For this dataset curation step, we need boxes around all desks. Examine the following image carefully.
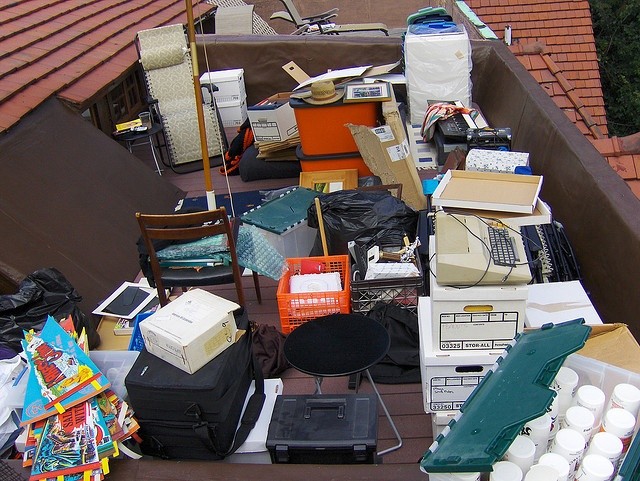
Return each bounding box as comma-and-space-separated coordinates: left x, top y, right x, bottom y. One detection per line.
282, 312, 402, 456
113, 118, 171, 177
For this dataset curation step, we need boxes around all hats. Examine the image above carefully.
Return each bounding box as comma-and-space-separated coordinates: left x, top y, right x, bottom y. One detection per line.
297, 80, 347, 104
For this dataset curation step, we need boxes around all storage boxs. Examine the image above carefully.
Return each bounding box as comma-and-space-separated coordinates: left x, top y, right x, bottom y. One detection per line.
350, 243, 425, 321
247, 90, 300, 143
417, 318, 640, 477
199, 68, 248, 103
430, 411, 462, 446
521, 280, 604, 331
140, 287, 246, 374
417, 296, 506, 412
229, 184, 325, 280
83, 351, 139, 404
292, 86, 388, 154
277, 255, 350, 336
300, 167, 358, 200
295, 143, 404, 177
524, 323, 640, 376
266, 394, 378, 465
429, 235, 528, 347
202, 98, 248, 126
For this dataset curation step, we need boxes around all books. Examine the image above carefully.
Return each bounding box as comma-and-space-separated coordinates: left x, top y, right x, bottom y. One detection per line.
18, 314, 142, 480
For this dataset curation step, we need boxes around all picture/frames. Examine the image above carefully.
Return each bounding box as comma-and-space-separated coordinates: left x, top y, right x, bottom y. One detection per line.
345, 81, 394, 102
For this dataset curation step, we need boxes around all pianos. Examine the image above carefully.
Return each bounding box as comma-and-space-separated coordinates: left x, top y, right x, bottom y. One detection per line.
427, 100, 478, 143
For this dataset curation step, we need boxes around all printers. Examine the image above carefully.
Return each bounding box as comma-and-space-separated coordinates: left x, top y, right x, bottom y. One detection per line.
435, 212, 579, 287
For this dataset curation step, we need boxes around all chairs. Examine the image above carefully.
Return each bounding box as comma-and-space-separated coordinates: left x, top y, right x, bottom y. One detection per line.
268, 0, 391, 38
133, 22, 228, 175
135, 205, 262, 311
